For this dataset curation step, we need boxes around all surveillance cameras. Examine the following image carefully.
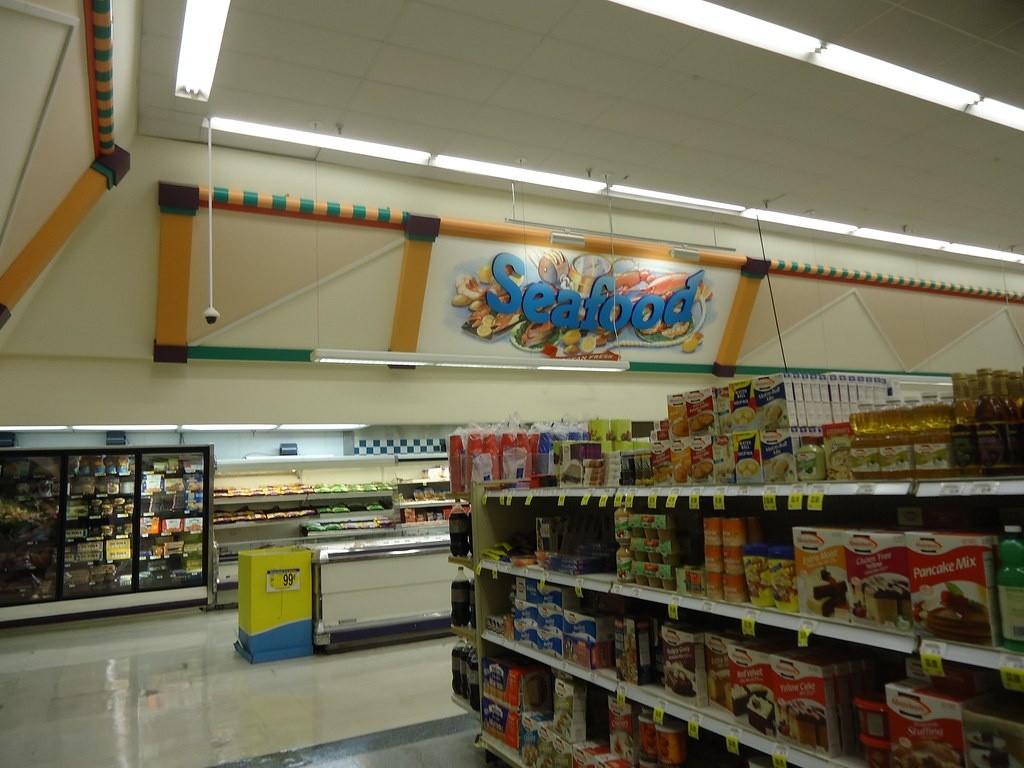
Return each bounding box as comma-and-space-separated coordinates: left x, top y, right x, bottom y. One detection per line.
203, 306, 220, 325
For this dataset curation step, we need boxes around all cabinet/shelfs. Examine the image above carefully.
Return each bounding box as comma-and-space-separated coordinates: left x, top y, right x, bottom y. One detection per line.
212, 453, 473, 609
442, 476, 1024, 768
0, 443, 215, 629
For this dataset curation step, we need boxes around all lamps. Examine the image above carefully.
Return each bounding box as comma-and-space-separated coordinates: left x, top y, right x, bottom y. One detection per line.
307, 146, 630, 374
201, 115, 1024, 266
174, 1, 233, 103
607, 0, 1024, 133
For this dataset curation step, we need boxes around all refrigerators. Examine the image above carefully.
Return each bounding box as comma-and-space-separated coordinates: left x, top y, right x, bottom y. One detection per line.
0, 444, 216, 629
301, 534, 451, 652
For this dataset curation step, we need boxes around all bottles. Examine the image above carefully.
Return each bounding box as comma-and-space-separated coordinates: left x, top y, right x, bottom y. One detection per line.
851, 397, 951, 480
950, 372, 977, 476
449, 498, 473, 557
622, 448, 654, 485
451, 567, 476, 630
997, 525, 1024, 653
975, 368, 1024, 476
452, 633, 480, 713
